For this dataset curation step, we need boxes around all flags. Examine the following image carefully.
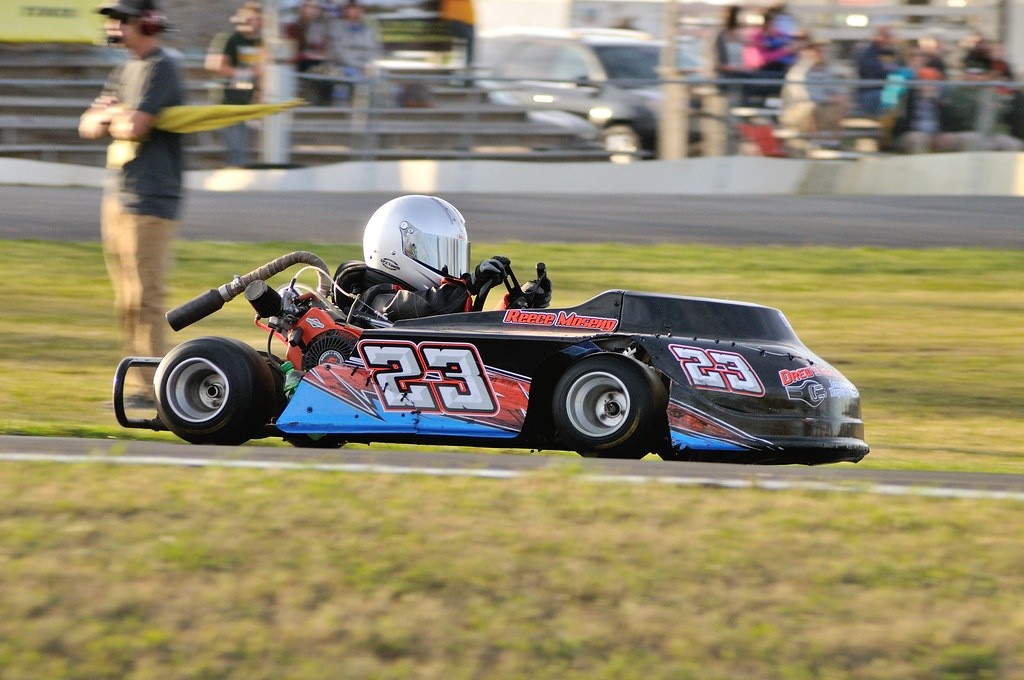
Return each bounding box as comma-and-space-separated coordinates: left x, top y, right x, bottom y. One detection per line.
152, 101, 292, 134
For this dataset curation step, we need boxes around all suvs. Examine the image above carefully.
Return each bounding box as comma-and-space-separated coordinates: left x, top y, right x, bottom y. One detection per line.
475, 28, 711, 148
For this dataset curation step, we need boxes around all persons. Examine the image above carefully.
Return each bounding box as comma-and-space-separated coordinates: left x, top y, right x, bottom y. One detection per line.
361, 193, 551, 320
286, 0, 330, 96
713, 0, 857, 148
205, 2, 265, 169
855, 27, 1013, 132
329, 0, 376, 106
78, 0, 187, 409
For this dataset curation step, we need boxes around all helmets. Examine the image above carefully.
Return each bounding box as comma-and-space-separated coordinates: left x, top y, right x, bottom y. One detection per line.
363, 193, 469, 292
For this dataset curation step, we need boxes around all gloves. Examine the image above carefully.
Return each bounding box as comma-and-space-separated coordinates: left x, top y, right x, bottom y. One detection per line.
462, 257, 510, 292
501, 275, 550, 310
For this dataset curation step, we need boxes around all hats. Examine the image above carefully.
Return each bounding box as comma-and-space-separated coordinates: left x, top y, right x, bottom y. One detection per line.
97, 0, 151, 16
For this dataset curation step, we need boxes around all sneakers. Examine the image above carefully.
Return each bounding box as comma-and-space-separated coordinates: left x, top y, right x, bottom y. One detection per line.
111, 393, 158, 410
